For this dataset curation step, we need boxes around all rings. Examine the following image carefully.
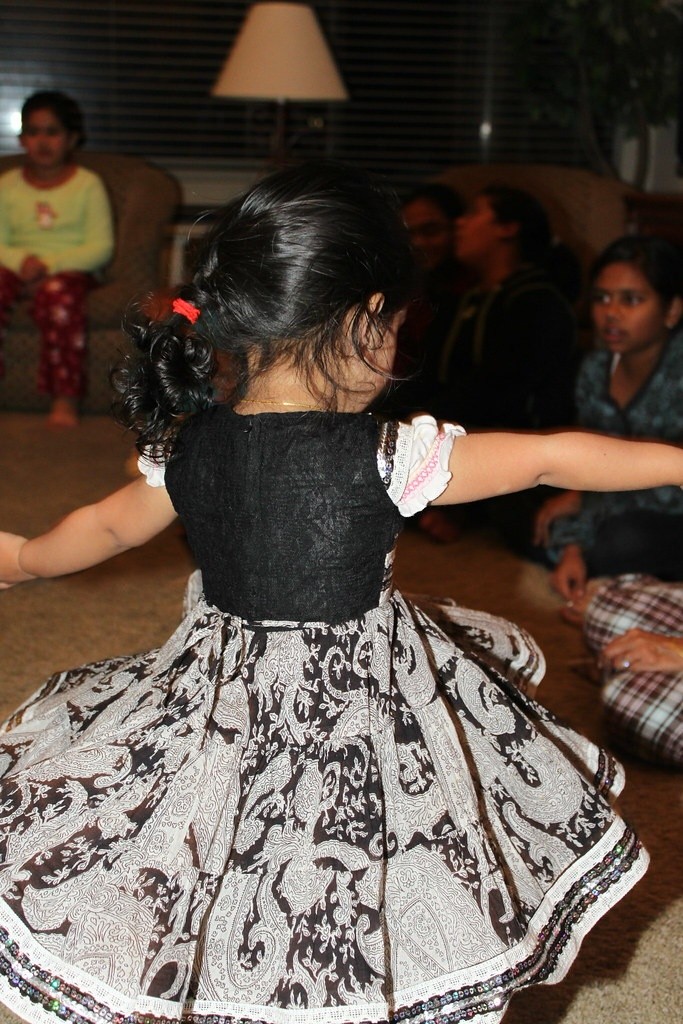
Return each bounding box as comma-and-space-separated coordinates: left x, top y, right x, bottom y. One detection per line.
622, 655, 629, 668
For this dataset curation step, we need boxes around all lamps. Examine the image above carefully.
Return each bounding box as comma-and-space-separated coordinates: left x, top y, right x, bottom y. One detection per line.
208, 1, 349, 169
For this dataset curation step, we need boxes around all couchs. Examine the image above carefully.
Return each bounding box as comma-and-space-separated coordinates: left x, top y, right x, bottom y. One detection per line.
0, 151, 180, 415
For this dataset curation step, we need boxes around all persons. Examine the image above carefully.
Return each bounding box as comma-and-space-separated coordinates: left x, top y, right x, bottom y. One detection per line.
0, 161, 682, 1024
353, 182, 683, 770
0, 91, 115, 432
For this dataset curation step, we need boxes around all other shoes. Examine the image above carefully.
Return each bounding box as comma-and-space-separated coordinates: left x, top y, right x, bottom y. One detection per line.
48, 410, 79, 428
